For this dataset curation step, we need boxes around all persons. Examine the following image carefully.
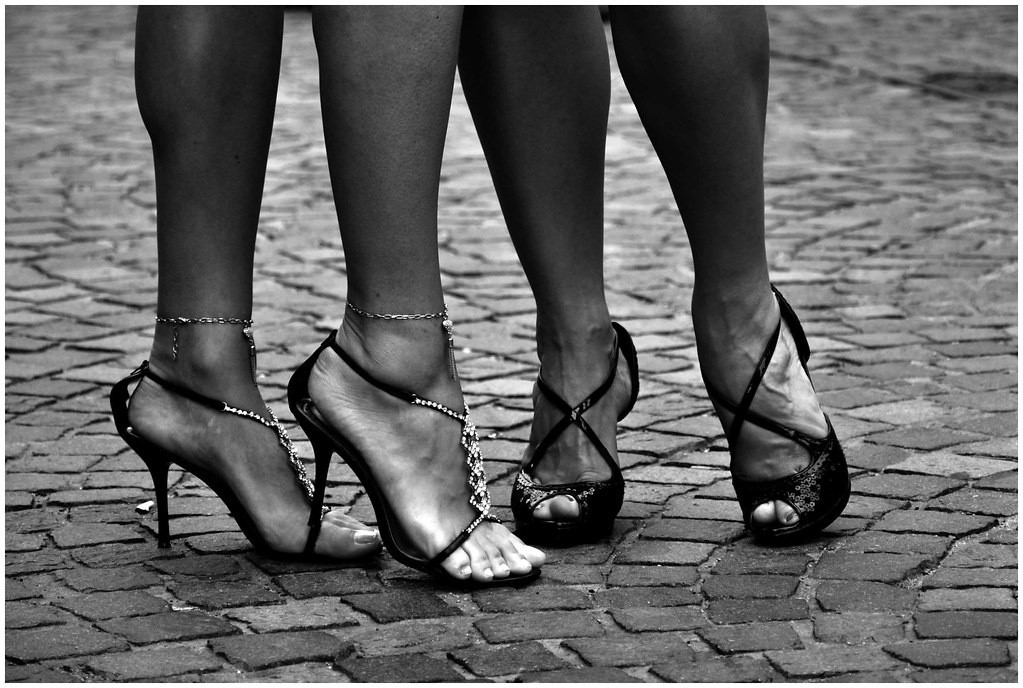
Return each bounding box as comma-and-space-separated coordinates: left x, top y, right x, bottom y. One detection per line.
107, 5, 546, 588
456, 5, 852, 545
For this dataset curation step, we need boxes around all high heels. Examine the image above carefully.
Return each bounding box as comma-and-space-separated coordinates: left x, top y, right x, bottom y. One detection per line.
701, 281, 851, 545
109, 350, 383, 562
287, 332, 541, 586
509, 322, 640, 545
